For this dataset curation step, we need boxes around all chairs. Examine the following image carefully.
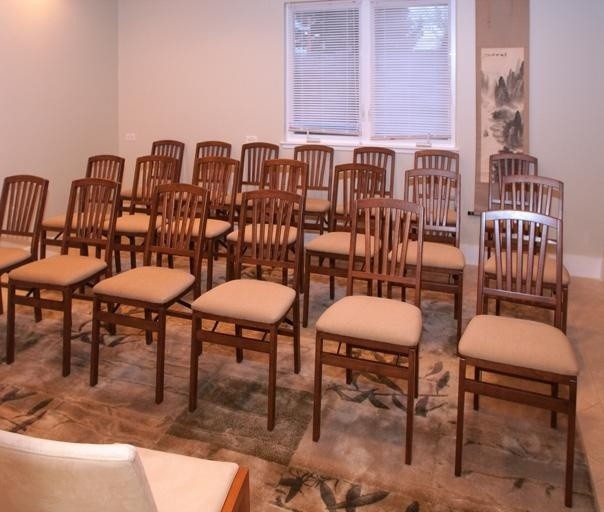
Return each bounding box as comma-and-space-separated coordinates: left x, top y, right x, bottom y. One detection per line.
41, 153, 124, 258
226, 158, 309, 293
388, 166, 466, 342
0, 430, 252, 512
0, 174, 50, 321
117, 139, 543, 254
303, 163, 386, 328
187, 190, 302, 432
310, 200, 427, 465
479, 173, 572, 333
156, 156, 244, 289
90, 183, 210, 404
455, 212, 578, 510
6, 178, 121, 377
95, 154, 180, 272
